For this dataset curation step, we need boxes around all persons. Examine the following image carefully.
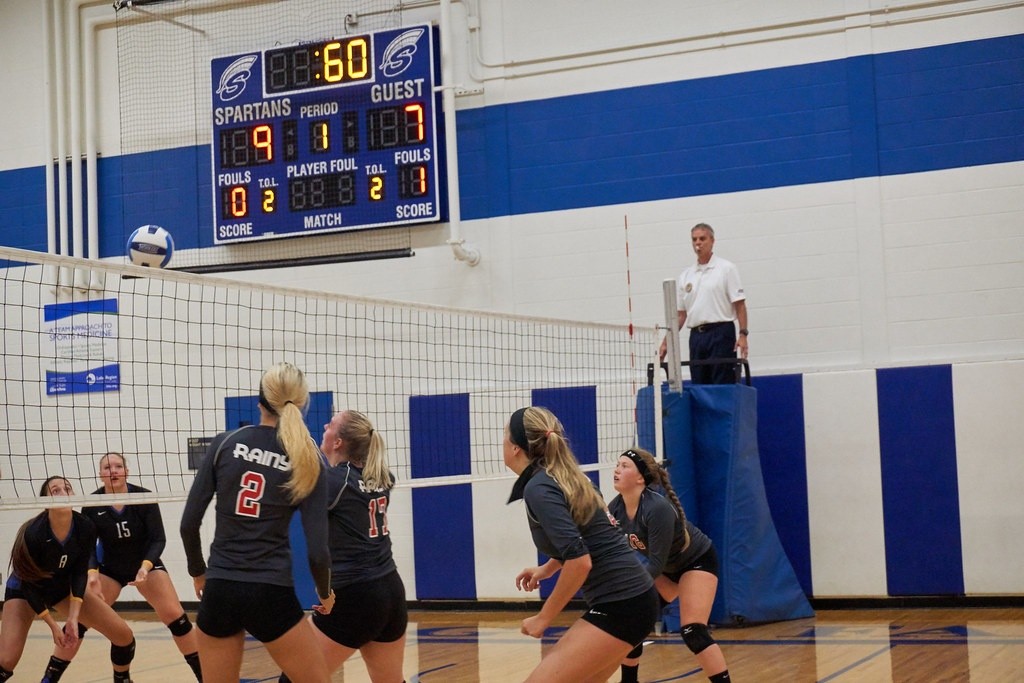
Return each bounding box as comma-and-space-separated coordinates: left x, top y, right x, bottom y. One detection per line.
180, 362, 335, 683
278, 410, 407, 683
504, 406, 660, 683
609, 449, 731, 683
659, 224, 748, 383
0, 475, 136, 683
40, 453, 202, 683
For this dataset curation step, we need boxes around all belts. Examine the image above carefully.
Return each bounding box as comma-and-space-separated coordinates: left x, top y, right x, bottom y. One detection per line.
692, 322, 733, 333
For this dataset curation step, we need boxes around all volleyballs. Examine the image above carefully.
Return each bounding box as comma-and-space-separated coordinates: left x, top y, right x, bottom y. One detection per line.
125, 224, 176, 270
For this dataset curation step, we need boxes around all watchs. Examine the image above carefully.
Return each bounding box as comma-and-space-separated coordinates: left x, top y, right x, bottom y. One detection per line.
740, 329, 748, 335
